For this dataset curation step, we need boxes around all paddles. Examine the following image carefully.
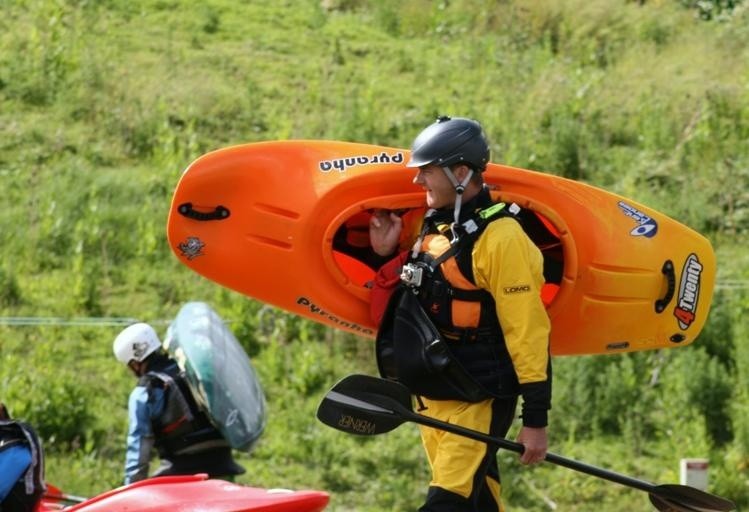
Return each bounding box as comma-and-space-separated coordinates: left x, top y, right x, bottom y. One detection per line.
42, 482, 88, 504
317, 374, 737, 512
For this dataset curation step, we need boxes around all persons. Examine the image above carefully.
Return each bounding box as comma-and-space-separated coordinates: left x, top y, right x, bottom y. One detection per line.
113, 322, 246, 486
365, 116, 553, 512
0, 402, 48, 511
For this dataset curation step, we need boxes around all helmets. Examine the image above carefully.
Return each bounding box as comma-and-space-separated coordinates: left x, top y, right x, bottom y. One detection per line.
112, 323, 163, 365
402, 115, 490, 173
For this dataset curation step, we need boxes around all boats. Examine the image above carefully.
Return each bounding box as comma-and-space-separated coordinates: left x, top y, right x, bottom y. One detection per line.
162, 301, 267, 450
165, 139, 717, 359
66, 473, 329, 512
